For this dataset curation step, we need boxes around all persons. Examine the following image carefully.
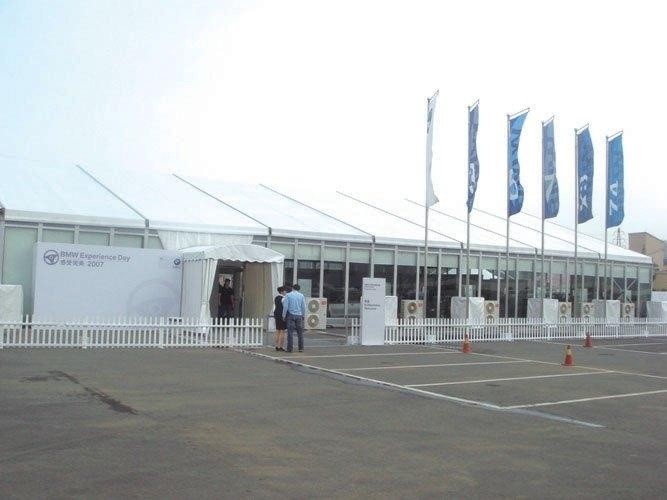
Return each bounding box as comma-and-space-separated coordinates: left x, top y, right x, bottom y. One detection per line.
273, 286, 288, 350
218, 278, 234, 330
281, 284, 305, 352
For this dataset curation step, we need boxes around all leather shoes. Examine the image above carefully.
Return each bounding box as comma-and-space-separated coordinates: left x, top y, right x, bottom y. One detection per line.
275, 346, 285, 351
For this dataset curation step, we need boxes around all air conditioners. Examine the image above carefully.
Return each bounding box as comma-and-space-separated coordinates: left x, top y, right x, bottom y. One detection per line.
619, 302, 635, 326
484, 301, 499, 328
399, 298, 426, 330
559, 301, 573, 328
304, 297, 327, 330
579, 302, 595, 326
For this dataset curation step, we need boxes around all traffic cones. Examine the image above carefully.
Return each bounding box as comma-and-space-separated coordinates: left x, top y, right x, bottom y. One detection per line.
583, 333, 593, 348
462, 334, 471, 353
562, 344, 573, 366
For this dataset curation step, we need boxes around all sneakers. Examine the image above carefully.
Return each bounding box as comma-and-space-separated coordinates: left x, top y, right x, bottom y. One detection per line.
284, 348, 291, 352
299, 349, 303, 352
